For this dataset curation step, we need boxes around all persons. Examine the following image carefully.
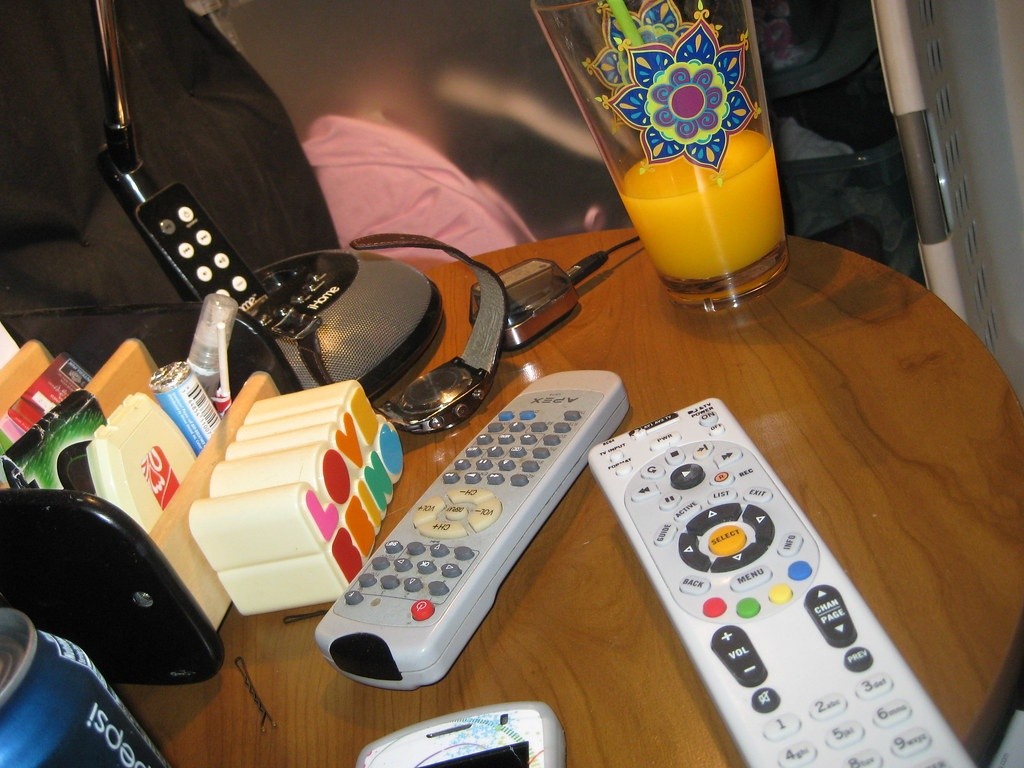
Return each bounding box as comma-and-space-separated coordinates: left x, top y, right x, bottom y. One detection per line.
0, 0, 929, 401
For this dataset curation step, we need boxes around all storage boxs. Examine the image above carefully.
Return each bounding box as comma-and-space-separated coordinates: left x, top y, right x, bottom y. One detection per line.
0, 303, 311, 687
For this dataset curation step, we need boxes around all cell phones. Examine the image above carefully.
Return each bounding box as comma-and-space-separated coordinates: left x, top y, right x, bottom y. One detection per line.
356, 699, 566, 768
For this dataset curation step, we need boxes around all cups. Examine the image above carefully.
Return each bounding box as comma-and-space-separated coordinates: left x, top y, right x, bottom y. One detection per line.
531, 0, 790, 314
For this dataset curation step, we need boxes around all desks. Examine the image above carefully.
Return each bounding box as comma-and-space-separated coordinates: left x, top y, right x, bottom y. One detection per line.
109, 227, 1024, 768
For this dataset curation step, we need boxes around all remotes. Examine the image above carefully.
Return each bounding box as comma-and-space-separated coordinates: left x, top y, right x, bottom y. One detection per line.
133, 180, 269, 317
313, 369, 634, 691
588, 398, 980, 768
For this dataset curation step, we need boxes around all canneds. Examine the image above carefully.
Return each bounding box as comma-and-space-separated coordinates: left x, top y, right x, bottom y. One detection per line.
0, 607, 175, 768
